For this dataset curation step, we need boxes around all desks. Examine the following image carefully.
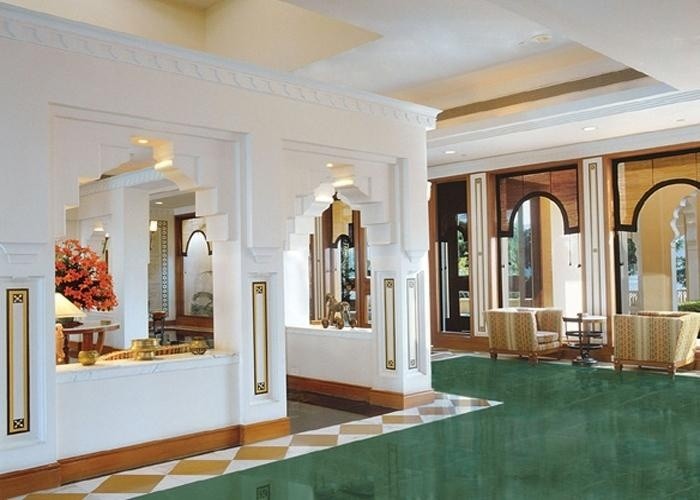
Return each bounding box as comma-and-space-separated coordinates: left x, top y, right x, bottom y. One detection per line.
63, 322, 120, 364
563, 314, 608, 367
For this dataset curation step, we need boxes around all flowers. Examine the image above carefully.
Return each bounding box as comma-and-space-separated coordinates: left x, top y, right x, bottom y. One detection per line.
55, 240, 119, 312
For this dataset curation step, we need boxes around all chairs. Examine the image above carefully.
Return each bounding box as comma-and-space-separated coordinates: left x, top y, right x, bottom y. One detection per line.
486, 307, 563, 364
614, 311, 700, 375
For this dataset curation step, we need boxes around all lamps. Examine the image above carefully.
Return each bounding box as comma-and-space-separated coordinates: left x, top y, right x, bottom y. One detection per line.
149, 221, 157, 250
54, 293, 88, 365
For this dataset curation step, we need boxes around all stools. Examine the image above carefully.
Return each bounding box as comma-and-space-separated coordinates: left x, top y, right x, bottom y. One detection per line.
152, 310, 167, 341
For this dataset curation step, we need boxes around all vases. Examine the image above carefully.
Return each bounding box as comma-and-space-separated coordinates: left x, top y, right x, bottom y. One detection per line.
56, 317, 73, 329
